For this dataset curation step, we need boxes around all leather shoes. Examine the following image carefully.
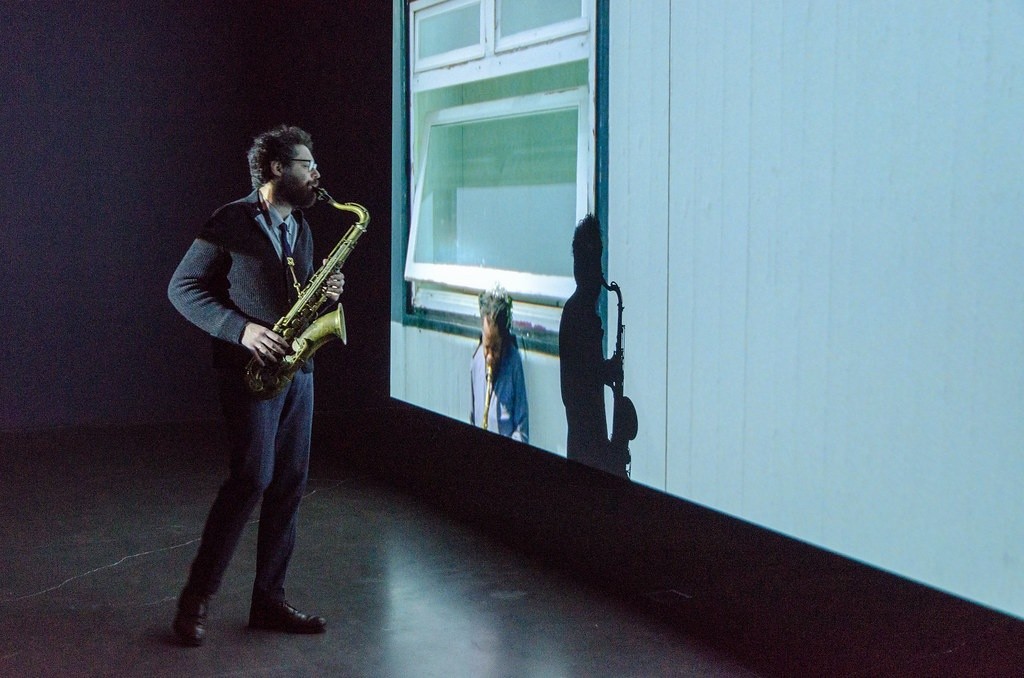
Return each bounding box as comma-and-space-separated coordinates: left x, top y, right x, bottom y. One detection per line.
172, 597, 209, 645
248, 604, 327, 633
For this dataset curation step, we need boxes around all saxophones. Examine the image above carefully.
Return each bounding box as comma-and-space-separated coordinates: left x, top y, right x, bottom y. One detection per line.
241, 187, 372, 402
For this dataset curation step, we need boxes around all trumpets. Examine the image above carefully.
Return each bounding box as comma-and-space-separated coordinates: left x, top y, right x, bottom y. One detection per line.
481, 355, 497, 431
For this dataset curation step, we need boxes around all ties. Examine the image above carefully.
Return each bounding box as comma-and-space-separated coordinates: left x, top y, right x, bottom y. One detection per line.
278, 222, 293, 263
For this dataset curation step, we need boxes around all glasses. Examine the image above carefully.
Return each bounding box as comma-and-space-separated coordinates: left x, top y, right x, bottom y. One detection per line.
288, 157, 318, 172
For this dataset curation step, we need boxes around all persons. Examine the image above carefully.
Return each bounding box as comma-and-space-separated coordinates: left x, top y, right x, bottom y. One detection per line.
166, 125, 347, 646
469, 284, 530, 444
